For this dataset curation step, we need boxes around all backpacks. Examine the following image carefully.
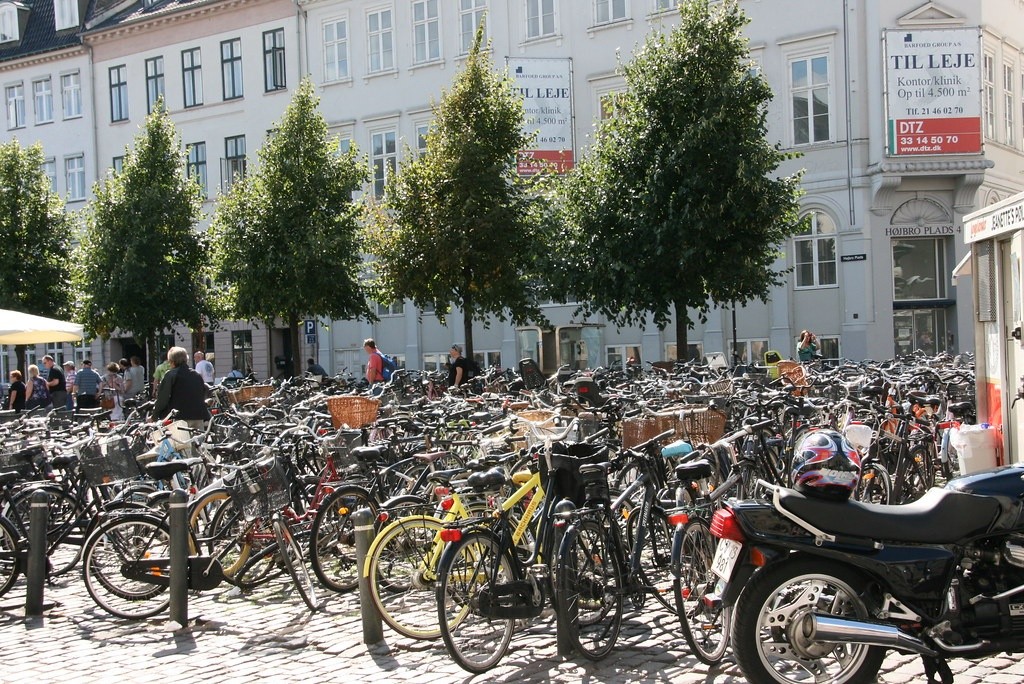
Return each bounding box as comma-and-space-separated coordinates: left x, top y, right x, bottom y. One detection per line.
373, 352, 398, 380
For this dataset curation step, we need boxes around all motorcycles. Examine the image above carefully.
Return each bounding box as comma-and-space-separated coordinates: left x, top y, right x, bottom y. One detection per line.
707, 379, 1023, 684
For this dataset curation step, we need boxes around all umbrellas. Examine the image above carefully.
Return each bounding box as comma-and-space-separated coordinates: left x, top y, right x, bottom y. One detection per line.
0, 309, 84, 345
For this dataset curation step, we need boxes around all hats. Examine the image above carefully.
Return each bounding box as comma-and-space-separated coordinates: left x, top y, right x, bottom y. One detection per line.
81, 360, 92, 366
62, 361, 74, 366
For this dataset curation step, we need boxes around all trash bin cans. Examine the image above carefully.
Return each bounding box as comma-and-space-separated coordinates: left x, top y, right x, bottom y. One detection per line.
950, 423, 997, 476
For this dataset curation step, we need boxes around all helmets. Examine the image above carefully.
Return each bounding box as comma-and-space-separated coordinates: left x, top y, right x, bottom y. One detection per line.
792, 429, 862, 500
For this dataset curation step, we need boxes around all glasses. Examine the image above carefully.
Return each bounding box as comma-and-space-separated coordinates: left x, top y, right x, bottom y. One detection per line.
451, 345, 457, 351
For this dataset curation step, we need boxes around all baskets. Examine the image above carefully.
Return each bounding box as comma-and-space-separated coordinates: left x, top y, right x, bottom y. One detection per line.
657, 403, 727, 449
227, 385, 272, 407
223, 453, 292, 523
622, 418, 658, 450
700, 380, 736, 396
327, 395, 378, 431
537, 441, 610, 507
79, 438, 141, 487
776, 361, 809, 396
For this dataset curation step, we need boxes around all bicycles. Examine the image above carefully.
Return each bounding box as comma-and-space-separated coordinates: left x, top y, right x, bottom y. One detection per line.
1, 353, 976, 677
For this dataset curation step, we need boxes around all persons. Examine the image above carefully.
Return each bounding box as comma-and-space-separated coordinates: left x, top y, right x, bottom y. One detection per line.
797, 330, 820, 396
147, 348, 207, 488
909, 328, 934, 353
305, 359, 328, 378
363, 338, 392, 438
444, 344, 468, 394
227, 364, 255, 382
194, 352, 214, 398
8, 357, 145, 428
